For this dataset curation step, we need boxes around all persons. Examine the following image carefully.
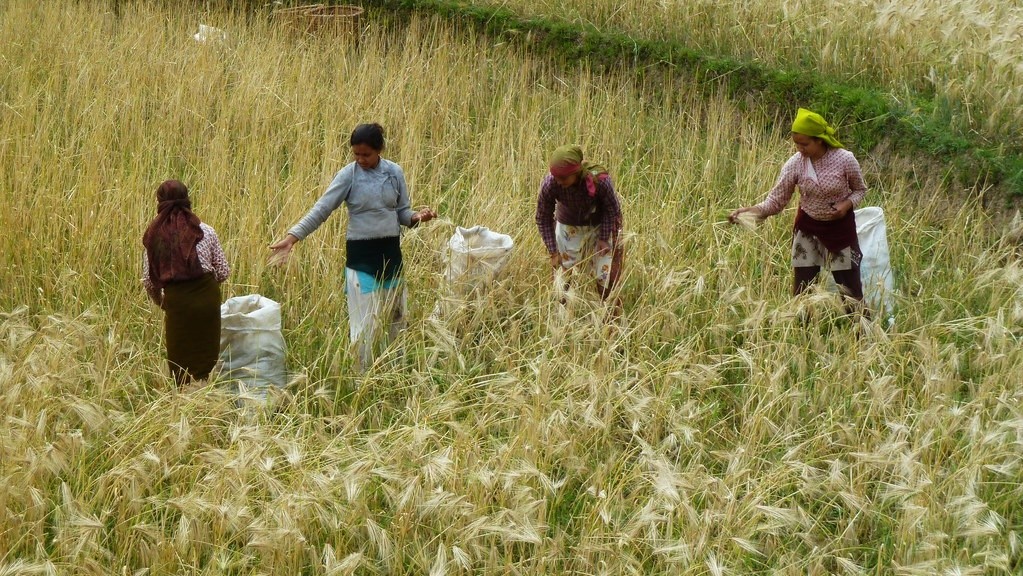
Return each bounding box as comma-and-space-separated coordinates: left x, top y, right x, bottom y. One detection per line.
729, 107, 870, 329
142, 180, 229, 387
535, 144, 626, 317
268, 122, 436, 368
